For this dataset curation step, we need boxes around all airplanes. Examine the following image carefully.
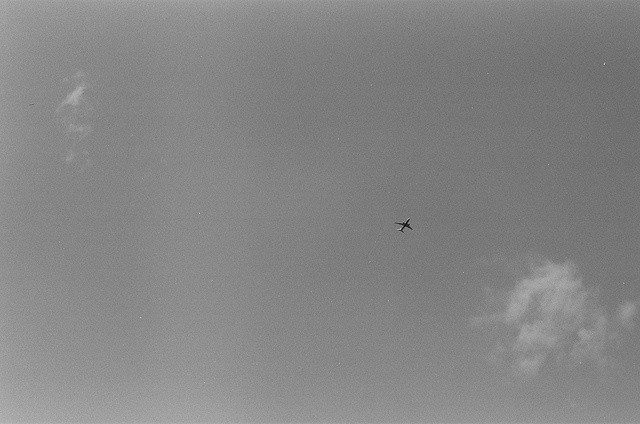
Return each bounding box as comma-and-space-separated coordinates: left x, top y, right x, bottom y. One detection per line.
395, 217, 413, 232
